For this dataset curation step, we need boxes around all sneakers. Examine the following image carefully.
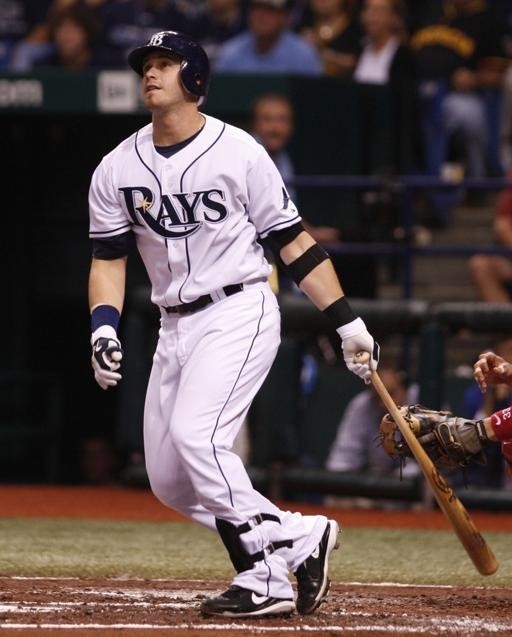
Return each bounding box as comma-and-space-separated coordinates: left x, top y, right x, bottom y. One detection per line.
295, 519, 340, 616
199, 585, 295, 618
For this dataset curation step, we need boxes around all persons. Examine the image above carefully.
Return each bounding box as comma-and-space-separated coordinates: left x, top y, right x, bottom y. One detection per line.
84, 30, 382, 618
2, 1, 512, 90
252, 45, 512, 510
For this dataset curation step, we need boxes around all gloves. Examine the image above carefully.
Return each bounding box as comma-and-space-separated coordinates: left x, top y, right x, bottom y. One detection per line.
90, 304, 125, 393
323, 297, 381, 385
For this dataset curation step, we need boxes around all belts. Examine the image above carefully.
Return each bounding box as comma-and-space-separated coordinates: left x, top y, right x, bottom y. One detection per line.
159, 282, 245, 318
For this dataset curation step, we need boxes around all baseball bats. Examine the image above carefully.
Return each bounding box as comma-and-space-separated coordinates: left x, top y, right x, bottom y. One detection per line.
354, 350, 500, 575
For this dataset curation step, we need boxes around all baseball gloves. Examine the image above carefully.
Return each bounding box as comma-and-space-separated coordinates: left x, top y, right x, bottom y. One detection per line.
379, 403, 501, 474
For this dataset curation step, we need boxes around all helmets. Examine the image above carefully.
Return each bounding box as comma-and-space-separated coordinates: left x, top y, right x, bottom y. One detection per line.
127, 28, 212, 108
